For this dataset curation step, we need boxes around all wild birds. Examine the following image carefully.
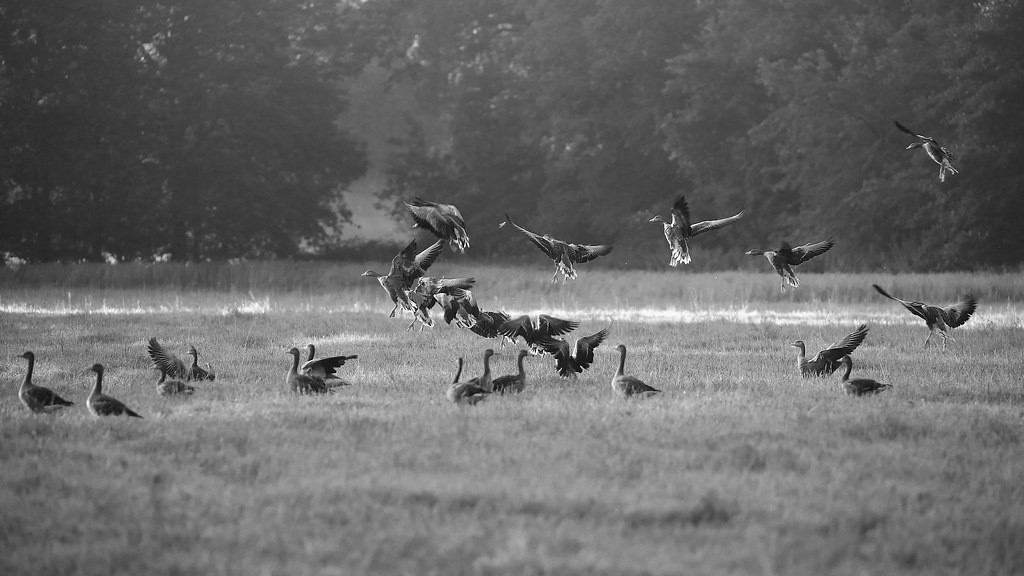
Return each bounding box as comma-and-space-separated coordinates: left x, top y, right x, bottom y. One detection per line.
788, 324, 870, 379
147, 335, 217, 384
498, 212, 616, 286
301, 343, 359, 389
836, 354, 894, 398
491, 349, 536, 395
15, 350, 76, 414
284, 346, 335, 394
744, 235, 836, 294
360, 195, 614, 380
445, 356, 493, 405
150, 362, 197, 397
648, 193, 745, 266
81, 363, 145, 419
467, 347, 502, 393
610, 343, 662, 398
872, 283, 978, 353
891, 117, 961, 183
